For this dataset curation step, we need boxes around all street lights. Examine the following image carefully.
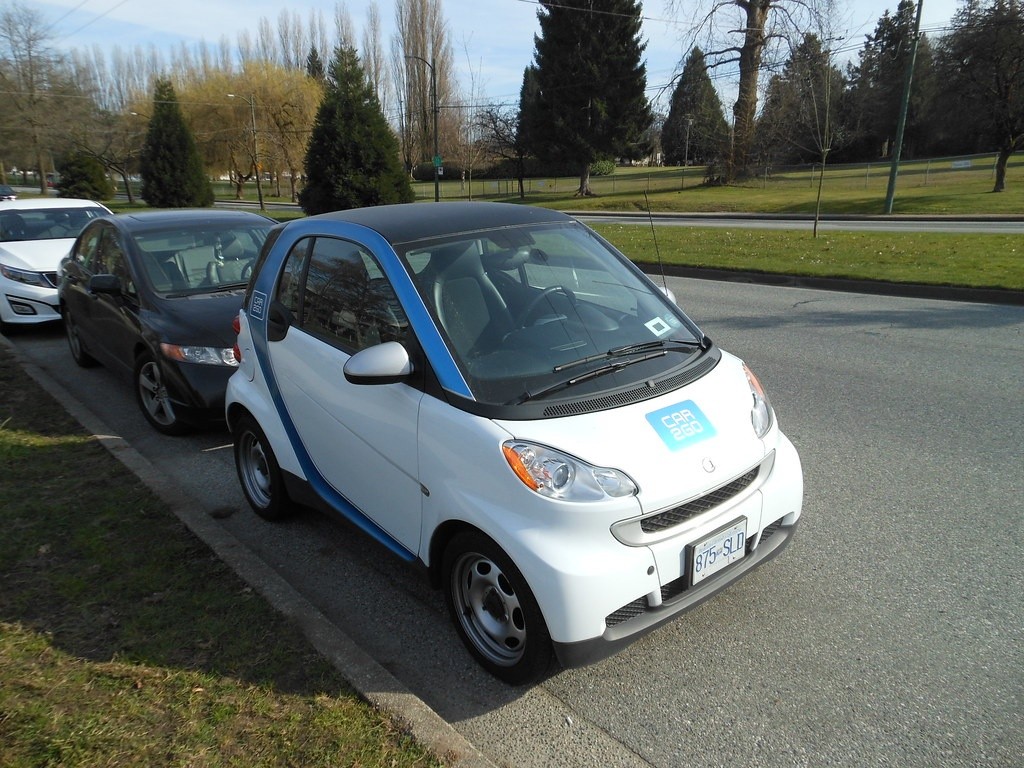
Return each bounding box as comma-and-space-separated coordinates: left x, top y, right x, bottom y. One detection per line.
402, 55, 437, 204
227, 94, 264, 210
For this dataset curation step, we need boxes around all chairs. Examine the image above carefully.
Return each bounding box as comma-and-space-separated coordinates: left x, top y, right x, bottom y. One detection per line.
101, 229, 258, 293
423, 240, 515, 363
305, 237, 401, 352
0, 212, 93, 240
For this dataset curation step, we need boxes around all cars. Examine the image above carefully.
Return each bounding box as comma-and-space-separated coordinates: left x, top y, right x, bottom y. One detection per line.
1, 196, 117, 326
223, 199, 804, 687
56, 208, 284, 431
